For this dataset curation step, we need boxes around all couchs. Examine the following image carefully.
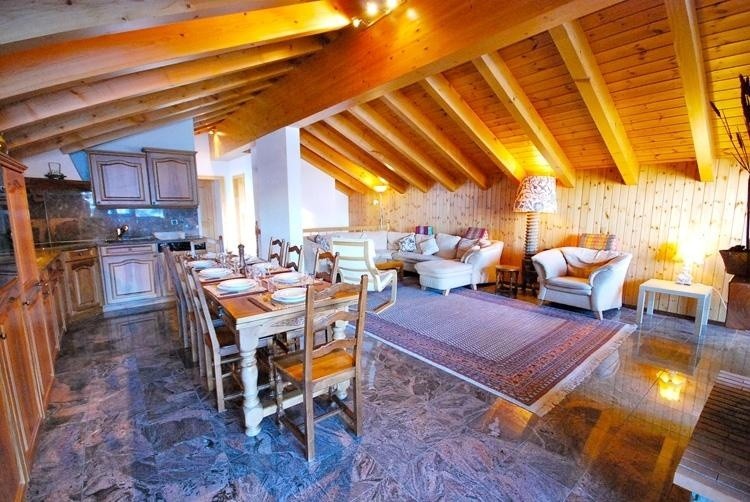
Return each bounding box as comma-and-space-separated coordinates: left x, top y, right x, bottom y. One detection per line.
305, 230, 503, 296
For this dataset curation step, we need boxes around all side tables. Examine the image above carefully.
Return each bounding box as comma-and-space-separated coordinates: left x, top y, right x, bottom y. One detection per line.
639, 279, 713, 336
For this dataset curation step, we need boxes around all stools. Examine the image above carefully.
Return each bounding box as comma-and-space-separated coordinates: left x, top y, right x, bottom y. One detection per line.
414, 261, 473, 296
496, 265, 520, 297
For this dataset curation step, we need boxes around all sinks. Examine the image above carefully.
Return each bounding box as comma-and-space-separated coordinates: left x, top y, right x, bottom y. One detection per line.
106, 236, 156, 244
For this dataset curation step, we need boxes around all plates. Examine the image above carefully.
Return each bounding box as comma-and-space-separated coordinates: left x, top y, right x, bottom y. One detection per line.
271, 272, 307, 303
233, 256, 257, 264
190, 260, 258, 291
200, 252, 219, 260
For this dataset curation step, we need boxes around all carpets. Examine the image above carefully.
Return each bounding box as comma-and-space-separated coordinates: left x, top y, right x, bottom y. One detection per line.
349, 275, 637, 416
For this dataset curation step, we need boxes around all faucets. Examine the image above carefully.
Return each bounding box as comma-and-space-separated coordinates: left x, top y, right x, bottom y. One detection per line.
116, 226, 129, 239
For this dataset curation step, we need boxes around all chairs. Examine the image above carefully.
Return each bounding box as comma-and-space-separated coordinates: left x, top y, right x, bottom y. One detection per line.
162, 247, 207, 349
267, 237, 285, 266
183, 260, 274, 414
312, 247, 340, 283
189, 236, 224, 254
269, 275, 366, 462
332, 238, 398, 315
283, 241, 304, 271
532, 247, 633, 321
170, 253, 221, 377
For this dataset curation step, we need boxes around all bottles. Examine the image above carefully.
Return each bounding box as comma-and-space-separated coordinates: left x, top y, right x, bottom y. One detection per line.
238, 244, 247, 272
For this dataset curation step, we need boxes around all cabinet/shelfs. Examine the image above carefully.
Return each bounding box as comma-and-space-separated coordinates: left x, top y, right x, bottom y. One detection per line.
141, 147, 198, 208
99, 246, 158, 314
0, 244, 101, 502
88, 150, 152, 207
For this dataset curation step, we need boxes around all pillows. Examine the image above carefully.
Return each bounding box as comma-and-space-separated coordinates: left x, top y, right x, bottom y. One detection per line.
369, 221, 492, 263
580, 233, 616, 250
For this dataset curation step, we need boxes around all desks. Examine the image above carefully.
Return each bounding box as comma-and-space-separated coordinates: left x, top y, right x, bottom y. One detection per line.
673, 370, 750, 502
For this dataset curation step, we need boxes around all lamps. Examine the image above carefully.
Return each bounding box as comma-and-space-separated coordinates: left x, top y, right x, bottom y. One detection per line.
514, 175, 557, 296
374, 186, 387, 230
